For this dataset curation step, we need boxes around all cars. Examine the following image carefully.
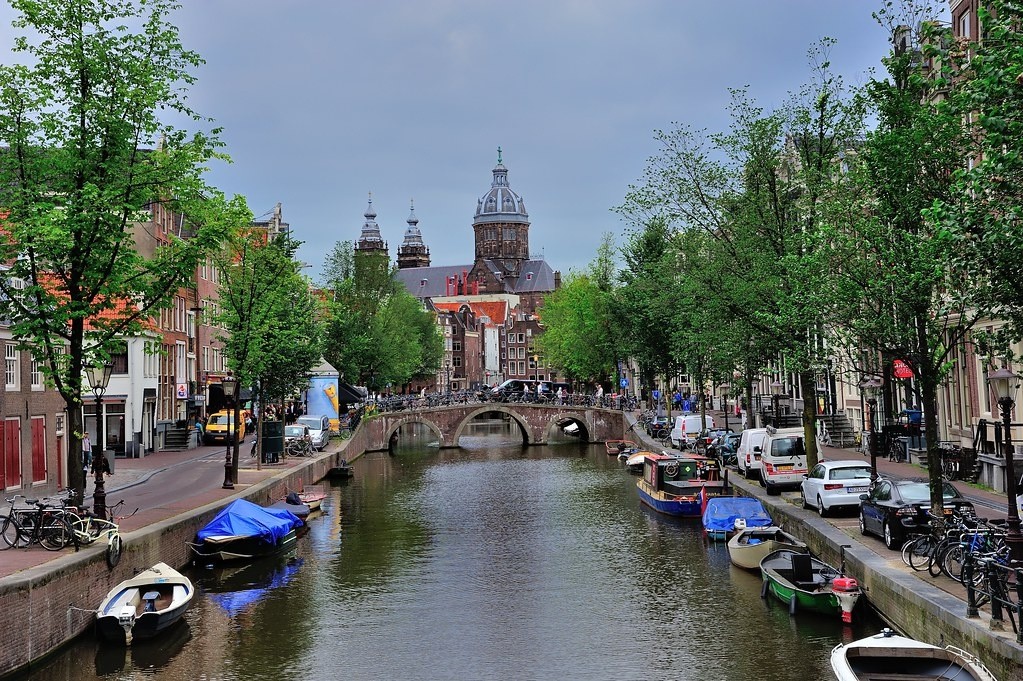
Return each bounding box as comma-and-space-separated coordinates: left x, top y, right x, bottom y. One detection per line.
220, 409, 255, 434
858, 475, 977, 553
695, 427, 741, 466
799, 460, 882, 517
285, 425, 311, 446
646, 415, 675, 439
205, 413, 246, 445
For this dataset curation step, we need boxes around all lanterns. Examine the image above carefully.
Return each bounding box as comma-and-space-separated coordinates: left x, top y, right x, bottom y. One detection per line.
486, 373, 490, 377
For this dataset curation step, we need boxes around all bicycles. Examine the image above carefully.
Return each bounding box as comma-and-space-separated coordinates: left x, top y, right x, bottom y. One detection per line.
863, 431, 911, 463
251, 432, 258, 457
286, 434, 320, 459
896, 500, 1023, 599
937, 444, 968, 483
0, 494, 140, 570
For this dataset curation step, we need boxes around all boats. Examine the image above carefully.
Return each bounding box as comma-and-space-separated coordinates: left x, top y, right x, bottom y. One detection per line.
602, 437, 732, 518
758, 547, 866, 619
720, 518, 810, 572
94, 561, 194, 646
333, 457, 356, 480
194, 497, 303, 568
829, 627, 997, 681
264, 478, 328, 524
701, 496, 778, 548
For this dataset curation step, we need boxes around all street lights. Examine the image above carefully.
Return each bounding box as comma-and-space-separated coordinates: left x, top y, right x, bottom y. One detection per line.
446, 362, 450, 406
217, 370, 244, 492
986, 365, 1023, 562
720, 382, 731, 436
82, 352, 120, 534
771, 379, 788, 434
861, 377, 883, 491
533, 353, 540, 401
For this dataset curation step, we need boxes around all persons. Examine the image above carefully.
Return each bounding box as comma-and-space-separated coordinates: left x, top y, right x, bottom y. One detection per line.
556, 387, 569, 406
674, 390, 683, 410
244, 401, 303, 435
595, 384, 603, 407
82, 432, 92, 467
522, 382, 530, 402
533, 381, 543, 403
421, 387, 426, 398
903, 405, 922, 422
196, 413, 210, 446
689, 394, 697, 412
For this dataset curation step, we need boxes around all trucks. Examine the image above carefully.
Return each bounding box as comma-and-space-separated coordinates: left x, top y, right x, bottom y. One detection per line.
670, 415, 716, 452
754, 424, 824, 496
735, 428, 766, 481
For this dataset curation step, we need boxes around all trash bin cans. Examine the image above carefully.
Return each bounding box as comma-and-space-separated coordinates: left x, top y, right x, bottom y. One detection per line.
102, 450, 114, 475
713, 396, 720, 410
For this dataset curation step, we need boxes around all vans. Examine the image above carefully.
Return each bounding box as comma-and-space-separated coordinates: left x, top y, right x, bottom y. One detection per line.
296, 414, 332, 450
489, 379, 555, 403
553, 383, 574, 404
